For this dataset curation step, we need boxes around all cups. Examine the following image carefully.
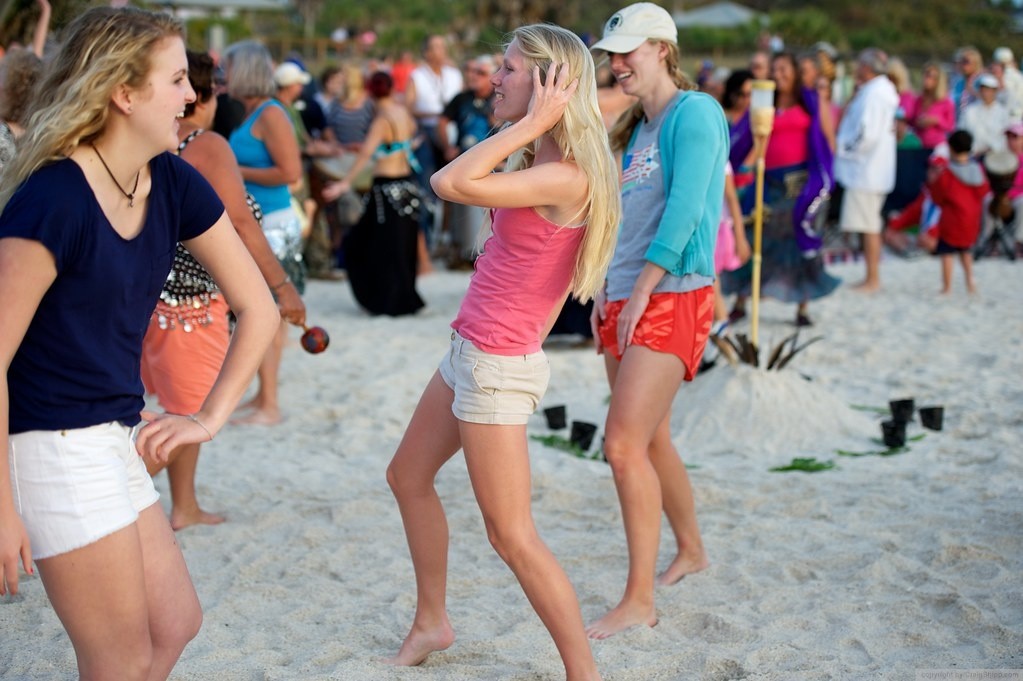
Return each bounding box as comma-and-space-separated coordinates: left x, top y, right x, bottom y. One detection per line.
544, 405, 566, 430
881, 398, 944, 449
571, 421, 598, 451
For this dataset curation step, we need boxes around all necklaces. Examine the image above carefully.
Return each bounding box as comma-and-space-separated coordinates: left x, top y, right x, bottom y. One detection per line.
90, 140, 139, 207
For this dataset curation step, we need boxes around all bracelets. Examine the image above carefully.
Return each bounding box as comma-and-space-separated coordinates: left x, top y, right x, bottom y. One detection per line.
190, 415, 214, 440
272, 277, 291, 290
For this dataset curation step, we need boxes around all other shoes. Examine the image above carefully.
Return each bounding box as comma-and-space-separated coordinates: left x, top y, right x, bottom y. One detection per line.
446, 257, 474, 270
727, 310, 744, 319
797, 313, 810, 326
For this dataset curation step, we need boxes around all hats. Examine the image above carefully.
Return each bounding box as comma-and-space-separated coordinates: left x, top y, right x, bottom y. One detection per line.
1004, 122, 1023, 136
925, 155, 949, 167
272, 62, 310, 86
590, 2, 677, 57
974, 74, 998, 89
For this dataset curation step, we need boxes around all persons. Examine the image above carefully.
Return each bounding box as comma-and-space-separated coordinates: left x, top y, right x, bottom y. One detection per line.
141, 50, 303, 530
586, 2, 729, 640
0, 8, 282, 680
386, 23, 619, 681
224, 39, 303, 426
0, 0, 1023, 341
